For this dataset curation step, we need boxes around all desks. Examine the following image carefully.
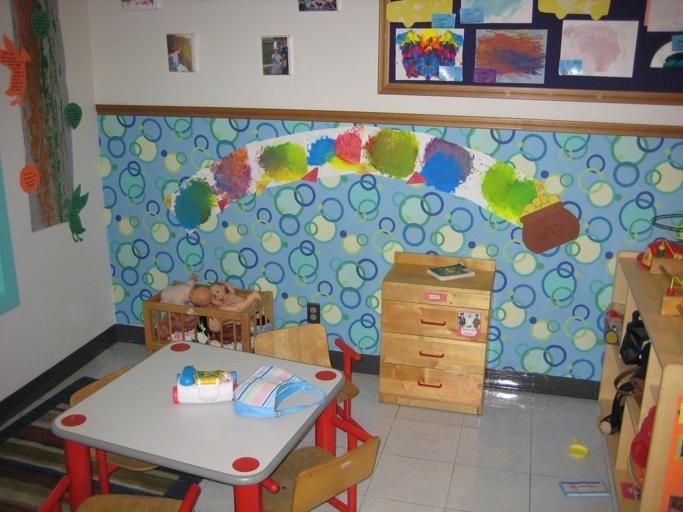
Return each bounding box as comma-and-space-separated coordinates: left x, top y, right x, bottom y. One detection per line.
49, 340, 345, 509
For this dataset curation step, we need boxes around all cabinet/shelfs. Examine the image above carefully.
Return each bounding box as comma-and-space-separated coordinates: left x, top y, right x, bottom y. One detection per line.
377, 249, 497, 418
599, 248, 683, 510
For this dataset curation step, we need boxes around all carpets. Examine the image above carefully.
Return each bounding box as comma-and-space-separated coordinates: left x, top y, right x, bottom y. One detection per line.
0, 373, 205, 511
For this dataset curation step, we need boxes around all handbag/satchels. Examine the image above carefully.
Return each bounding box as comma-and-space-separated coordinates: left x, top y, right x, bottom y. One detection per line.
599, 392, 625, 434
613, 366, 645, 408
232, 363, 327, 421
619, 310, 652, 365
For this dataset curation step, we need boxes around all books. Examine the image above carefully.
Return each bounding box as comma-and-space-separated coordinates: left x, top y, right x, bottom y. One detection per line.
426, 264, 475, 282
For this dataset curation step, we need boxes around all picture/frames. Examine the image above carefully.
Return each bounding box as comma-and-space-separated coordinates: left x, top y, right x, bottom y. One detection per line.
120, 1, 160, 12
297, 0, 341, 14
162, 31, 200, 76
373, 0, 682, 108
257, 32, 294, 80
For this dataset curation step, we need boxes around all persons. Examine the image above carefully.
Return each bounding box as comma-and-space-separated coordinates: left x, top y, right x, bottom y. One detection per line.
155, 273, 262, 346
272, 48, 283, 75
169, 48, 182, 71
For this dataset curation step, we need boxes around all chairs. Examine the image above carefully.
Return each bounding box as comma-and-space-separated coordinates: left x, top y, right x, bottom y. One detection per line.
261, 415, 379, 510
255, 324, 361, 425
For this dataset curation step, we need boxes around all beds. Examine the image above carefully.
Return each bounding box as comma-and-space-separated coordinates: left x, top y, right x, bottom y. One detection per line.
140, 280, 275, 357
